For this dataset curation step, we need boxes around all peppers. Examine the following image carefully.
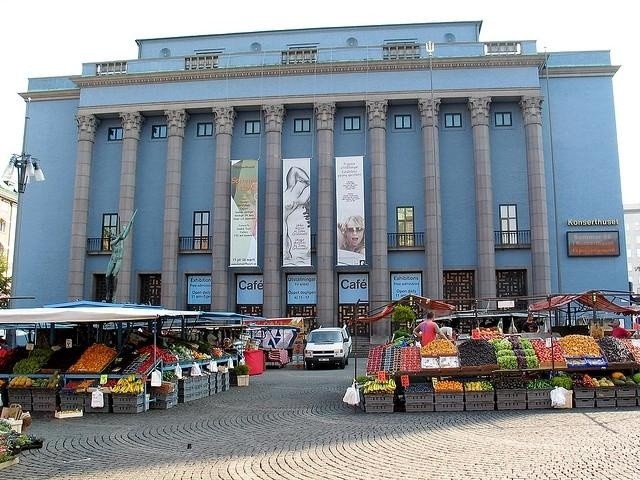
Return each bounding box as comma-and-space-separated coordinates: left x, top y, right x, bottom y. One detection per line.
526, 378, 553, 389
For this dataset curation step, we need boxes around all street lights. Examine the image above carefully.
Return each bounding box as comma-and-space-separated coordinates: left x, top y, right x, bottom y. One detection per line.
3, 152, 46, 309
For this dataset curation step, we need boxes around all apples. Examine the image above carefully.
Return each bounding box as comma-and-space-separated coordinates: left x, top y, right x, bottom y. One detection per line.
9, 376, 35, 388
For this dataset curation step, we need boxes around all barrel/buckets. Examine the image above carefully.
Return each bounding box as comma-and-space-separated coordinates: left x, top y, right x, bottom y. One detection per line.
242, 348, 264, 375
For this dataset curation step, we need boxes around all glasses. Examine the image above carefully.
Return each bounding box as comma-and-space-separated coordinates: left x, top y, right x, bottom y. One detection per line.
346, 228, 364, 232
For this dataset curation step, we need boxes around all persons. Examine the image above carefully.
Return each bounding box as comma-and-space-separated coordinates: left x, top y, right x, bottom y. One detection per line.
282, 167, 310, 259
440, 326, 458, 341
609, 318, 632, 338
338, 215, 365, 254
207, 330, 235, 348
413, 311, 448, 347
104, 208, 140, 303
342, 320, 347, 329
521, 312, 540, 334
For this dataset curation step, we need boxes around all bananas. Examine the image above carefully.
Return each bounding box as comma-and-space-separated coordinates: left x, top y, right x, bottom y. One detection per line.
363, 378, 397, 395
111, 375, 144, 394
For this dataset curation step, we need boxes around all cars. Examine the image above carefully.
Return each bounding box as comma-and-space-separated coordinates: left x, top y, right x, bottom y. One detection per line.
303, 324, 353, 370
